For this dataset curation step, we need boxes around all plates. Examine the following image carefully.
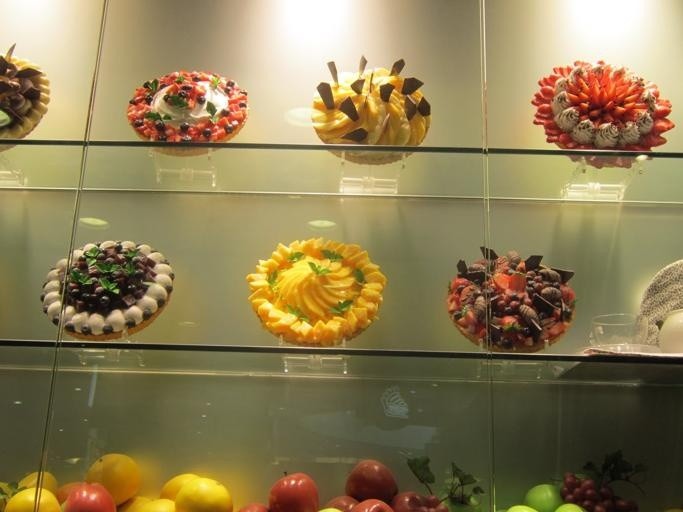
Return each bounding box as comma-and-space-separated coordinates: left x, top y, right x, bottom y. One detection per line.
582, 343, 683, 361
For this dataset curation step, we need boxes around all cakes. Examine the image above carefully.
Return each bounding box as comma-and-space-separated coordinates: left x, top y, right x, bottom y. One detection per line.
0, 42, 50, 152
312, 56, 432, 164
531, 61, 675, 169
40, 241, 175, 340
246, 237, 387, 347
127, 72, 248, 156
635, 259, 683, 348
447, 246, 576, 353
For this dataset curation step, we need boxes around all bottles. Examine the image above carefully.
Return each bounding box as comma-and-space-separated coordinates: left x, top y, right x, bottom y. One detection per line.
658, 309, 683, 351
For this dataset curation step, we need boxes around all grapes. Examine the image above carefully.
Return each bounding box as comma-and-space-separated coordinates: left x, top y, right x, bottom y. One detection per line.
561, 472, 639, 512
410, 494, 448, 512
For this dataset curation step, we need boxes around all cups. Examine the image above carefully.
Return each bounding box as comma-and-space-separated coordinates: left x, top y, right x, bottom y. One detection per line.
588, 313, 636, 350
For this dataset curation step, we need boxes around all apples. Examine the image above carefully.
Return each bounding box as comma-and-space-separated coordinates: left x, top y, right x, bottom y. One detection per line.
507, 485, 586, 512
238, 460, 414, 512
